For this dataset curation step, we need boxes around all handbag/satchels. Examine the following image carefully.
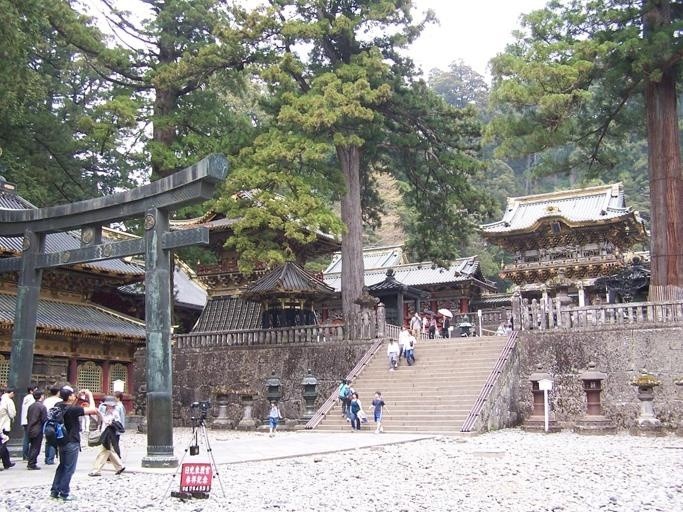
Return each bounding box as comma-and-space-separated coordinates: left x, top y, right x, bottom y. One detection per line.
337, 395, 346, 402
87, 430, 101, 447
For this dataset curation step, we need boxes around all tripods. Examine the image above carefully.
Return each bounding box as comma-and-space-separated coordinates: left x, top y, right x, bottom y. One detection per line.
160, 417, 228, 500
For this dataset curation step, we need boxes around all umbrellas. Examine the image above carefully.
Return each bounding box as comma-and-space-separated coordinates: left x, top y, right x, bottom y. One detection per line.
438, 308, 454, 318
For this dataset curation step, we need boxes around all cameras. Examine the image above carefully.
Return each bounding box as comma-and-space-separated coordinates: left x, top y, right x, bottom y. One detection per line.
191, 400, 212, 410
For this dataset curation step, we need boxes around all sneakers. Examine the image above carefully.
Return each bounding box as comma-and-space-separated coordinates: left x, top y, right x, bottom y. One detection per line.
87, 473, 101, 477
49, 493, 72, 502
27, 466, 40, 470
115, 467, 125, 475
4, 463, 15, 469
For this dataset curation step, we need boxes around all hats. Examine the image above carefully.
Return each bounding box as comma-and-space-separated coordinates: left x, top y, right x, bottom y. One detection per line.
101, 396, 118, 407
60, 385, 77, 396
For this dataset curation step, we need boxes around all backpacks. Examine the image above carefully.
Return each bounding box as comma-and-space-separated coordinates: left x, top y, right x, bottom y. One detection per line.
44, 404, 74, 447
343, 389, 352, 402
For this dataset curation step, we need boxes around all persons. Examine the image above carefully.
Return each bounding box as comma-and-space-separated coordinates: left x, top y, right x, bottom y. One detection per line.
387, 312, 450, 370
0, 385, 127, 501
267, 400, 282, 437
338, 378, 389, 433
